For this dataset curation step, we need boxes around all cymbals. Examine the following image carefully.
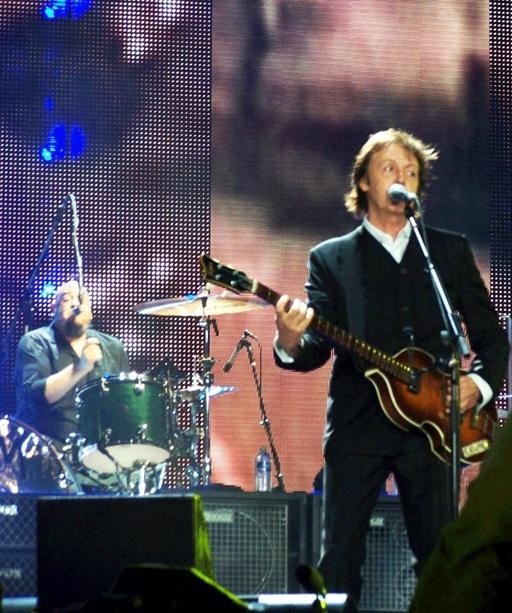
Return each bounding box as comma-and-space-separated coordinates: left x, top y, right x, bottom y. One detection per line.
168, 387, 236, 399
135, 295, 268, 316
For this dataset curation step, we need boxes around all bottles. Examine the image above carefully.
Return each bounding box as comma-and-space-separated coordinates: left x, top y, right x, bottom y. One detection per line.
255, 447, 272, 493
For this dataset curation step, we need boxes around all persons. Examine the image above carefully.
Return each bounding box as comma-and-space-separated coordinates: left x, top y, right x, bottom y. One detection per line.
13, 280, 131, 441
271, 124, 511, 611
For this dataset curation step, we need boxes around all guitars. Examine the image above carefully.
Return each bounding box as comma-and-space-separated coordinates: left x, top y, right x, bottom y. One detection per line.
198, 250, 499, 466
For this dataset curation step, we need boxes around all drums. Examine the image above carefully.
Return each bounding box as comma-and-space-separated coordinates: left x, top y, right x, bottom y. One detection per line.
73, 372, 169, 470
62, 430, 122, 486
0, 417, 80, 498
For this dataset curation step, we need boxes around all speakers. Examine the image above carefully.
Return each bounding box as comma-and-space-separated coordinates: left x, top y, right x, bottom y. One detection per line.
0, 492, 78, 600
158, 490, 307, 601
311, 492, 418, 613
34, 495, 216, 613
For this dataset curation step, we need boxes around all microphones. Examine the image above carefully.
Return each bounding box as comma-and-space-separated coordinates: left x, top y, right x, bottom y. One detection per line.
224, 336, 247, 373
297, 563, 327, 599
388, 183, 418, 203
72, 307, 83, 315
